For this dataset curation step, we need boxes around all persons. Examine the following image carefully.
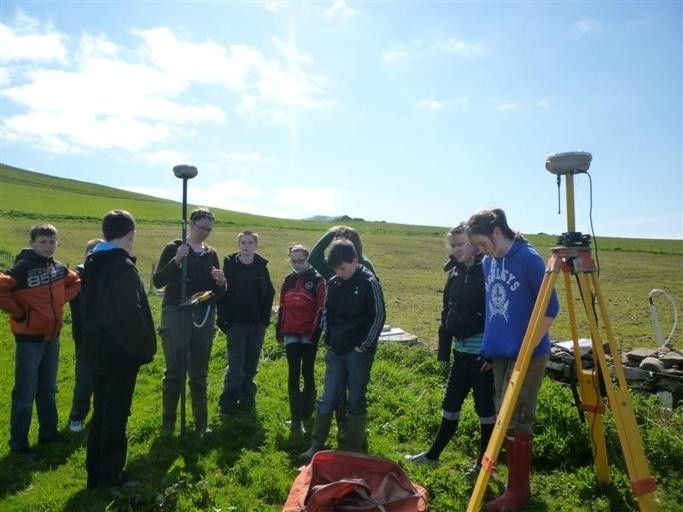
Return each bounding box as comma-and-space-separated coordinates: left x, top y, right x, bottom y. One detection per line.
152, 207, 228, 440
297, 237, 387, 462
0, 223, 82, 459
215, 230, 276, 416
68, 238, 107, 432
403, 221, 494, 481
78, 208, 157, 491
464, 208, 560, 511
274, 244, 329, 441
308, 225, 381, 451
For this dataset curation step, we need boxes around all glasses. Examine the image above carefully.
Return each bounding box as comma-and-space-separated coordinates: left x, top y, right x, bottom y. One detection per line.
195, 221, 210, 234
288, 259, 305, 264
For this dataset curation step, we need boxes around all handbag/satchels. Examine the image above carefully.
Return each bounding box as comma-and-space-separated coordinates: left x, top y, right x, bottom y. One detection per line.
283, 450, 429, 511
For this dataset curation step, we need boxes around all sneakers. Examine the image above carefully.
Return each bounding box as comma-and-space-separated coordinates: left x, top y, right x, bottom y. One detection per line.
11, 446, 40, 460
465, 463, 498, 476
403, 452, 440, 465
70, 420, 82, 432
38, 432, 72, 445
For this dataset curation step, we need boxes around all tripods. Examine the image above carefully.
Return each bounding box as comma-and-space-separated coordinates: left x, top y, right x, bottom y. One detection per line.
459, 235, 667, 512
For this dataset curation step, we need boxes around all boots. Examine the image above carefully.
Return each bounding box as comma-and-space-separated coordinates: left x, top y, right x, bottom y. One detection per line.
345, 413, 366, 452
484, 434, 530, 512
299, 412, 332, 462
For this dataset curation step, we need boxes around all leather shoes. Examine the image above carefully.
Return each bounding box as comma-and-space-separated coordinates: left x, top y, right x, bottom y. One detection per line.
88, 472, 128, 487
